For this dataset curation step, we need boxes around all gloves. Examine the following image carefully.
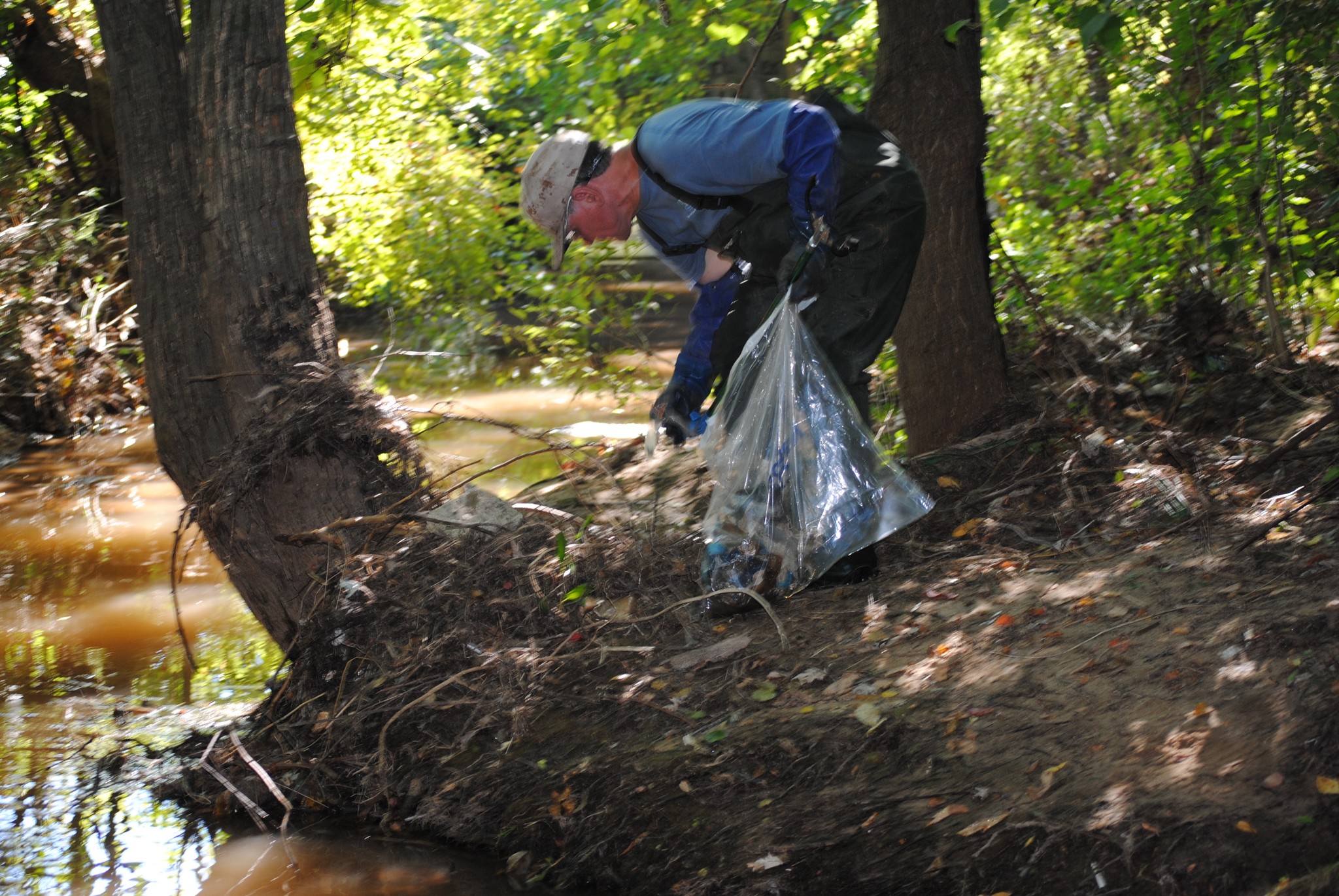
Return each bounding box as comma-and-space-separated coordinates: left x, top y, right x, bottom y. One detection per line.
651, 389, 697, 443
774, 240, 828, 301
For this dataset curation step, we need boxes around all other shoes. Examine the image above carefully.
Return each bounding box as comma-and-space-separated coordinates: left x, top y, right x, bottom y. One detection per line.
826, 548, 878, 582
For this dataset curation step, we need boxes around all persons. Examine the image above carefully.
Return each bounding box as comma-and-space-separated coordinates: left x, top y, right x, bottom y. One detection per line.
520, 84, 928, 590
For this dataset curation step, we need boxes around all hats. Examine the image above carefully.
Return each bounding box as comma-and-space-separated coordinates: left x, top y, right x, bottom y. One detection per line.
522, 130, 591, 269
